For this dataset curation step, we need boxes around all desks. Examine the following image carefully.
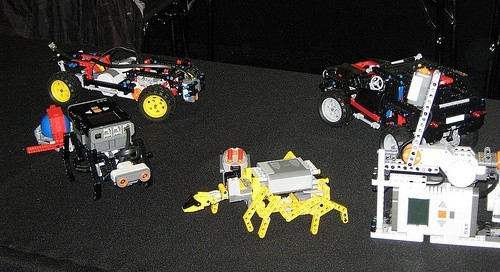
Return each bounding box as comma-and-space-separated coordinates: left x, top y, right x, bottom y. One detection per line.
1, 36, 500, 271
